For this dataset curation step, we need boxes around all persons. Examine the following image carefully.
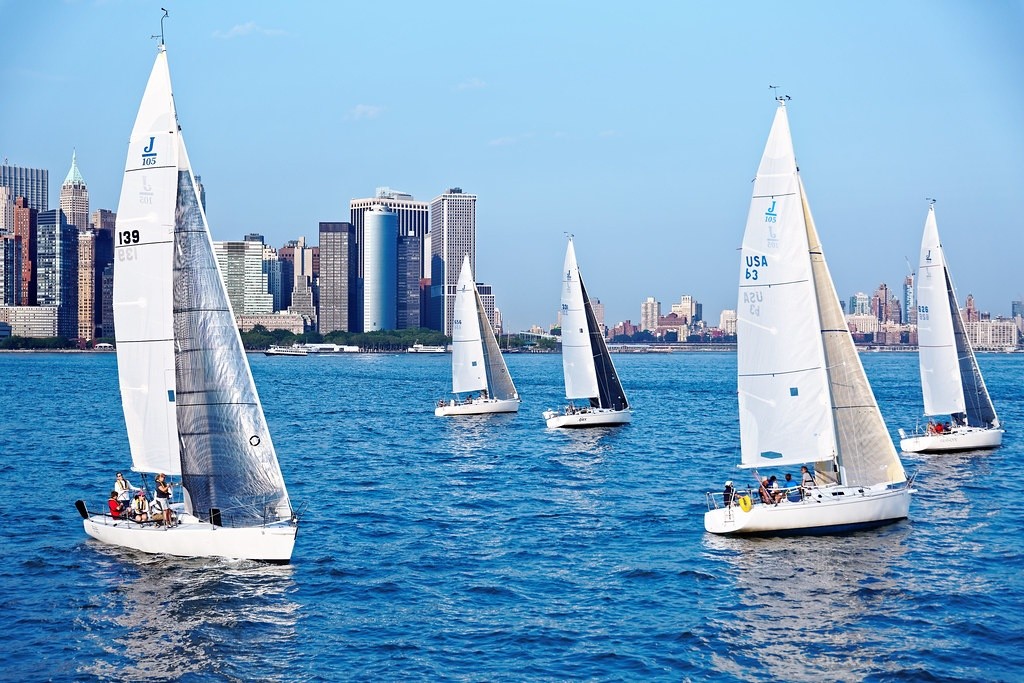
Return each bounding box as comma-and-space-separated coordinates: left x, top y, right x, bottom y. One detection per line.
108, 473, 173, 528
926, 421, 951, 436
758, 473, 803, 504
566, 403, 587, 415
723, 481, 744, 506
801, 466, 817, 489
437, 399, 444, 407
466, 395, 472, 404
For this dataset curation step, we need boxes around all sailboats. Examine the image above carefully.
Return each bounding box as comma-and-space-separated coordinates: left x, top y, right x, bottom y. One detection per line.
434, 252, 520, 416
542, 231, 631, 428
704, 85, 918, 533
74, 7, 309, 559
898, 198, 1007, 454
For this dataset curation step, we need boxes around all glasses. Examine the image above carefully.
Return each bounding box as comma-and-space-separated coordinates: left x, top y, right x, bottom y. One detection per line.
118, 474, 123, 477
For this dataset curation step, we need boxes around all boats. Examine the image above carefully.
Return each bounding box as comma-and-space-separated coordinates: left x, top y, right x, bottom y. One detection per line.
262, 340, 307, 356
408, 339, 445, 353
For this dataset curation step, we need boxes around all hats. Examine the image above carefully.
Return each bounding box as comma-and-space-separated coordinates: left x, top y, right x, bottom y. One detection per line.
762, 476, 770, 481
725, 481, 733, 486
138, 491, 145, 495
